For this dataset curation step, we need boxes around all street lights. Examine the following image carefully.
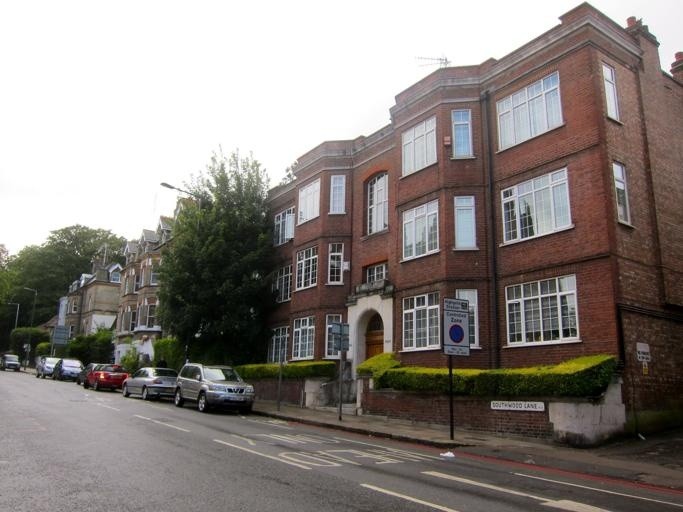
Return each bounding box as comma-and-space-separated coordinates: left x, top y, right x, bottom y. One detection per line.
24, 287, 38, 370
44, 325, 56, 357
8, 302, 20, 354
160, 179, 203, 238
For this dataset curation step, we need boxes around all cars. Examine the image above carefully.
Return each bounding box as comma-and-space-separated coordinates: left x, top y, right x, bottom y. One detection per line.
52, 358, 86, 382
84, 364, 132, 391
35, 355, 62, 379
122, 366, 179, 400
77, 363, 109, 387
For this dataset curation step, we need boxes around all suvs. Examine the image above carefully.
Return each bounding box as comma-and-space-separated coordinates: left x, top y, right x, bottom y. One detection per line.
0, 354, 21, 371
173, 363, 255, 415
